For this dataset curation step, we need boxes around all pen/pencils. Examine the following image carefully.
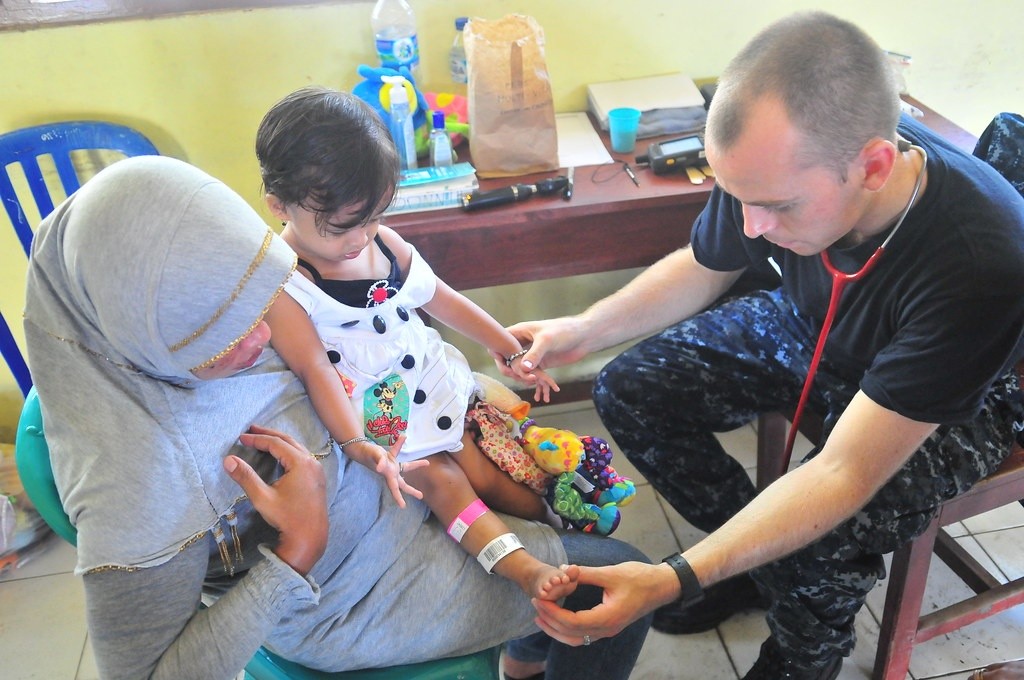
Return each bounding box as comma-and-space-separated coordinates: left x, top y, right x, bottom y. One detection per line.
566, 166, 575, 199
624, 165, 640, 188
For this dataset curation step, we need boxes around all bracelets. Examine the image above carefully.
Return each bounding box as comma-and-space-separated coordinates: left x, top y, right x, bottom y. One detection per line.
338, 436, 369, 450
506, 349, 528, 366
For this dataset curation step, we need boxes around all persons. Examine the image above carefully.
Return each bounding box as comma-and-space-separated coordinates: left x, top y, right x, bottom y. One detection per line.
490, 11, 1024, 680
256, 87, 580, 603
24, 155, 657, 680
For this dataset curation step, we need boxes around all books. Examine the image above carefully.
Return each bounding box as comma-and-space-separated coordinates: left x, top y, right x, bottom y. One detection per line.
380, 162, 479, 218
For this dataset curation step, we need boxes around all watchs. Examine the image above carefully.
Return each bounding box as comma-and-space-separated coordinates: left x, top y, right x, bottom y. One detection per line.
660, 552, 706, 612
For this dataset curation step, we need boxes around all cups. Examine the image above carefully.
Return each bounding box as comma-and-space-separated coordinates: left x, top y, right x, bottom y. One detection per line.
607, 107, 641, 154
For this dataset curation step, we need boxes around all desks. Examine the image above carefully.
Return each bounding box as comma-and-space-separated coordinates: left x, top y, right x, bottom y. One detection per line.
258, 94, 978, 408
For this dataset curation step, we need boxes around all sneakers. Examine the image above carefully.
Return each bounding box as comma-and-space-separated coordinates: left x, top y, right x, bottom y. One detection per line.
743, 631, 842, 680
650, 572, 766, 634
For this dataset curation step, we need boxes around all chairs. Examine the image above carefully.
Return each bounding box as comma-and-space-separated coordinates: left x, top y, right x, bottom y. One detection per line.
0, 121, 167, 392
754, 113, 1024, 680
18, 387, 499, 680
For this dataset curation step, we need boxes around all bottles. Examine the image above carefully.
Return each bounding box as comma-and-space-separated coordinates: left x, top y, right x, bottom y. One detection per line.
428, 111, 455, 167
449, 17, 470, 84
370, 0, 424, 93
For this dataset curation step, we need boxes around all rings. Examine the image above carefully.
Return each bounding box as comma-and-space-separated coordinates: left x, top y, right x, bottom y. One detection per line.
584, 636, 590, 646
399, 463, 405, 473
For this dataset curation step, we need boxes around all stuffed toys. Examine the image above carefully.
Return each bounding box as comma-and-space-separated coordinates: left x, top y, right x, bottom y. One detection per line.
354, 65, 428, 160
506, 414, 636, 537
